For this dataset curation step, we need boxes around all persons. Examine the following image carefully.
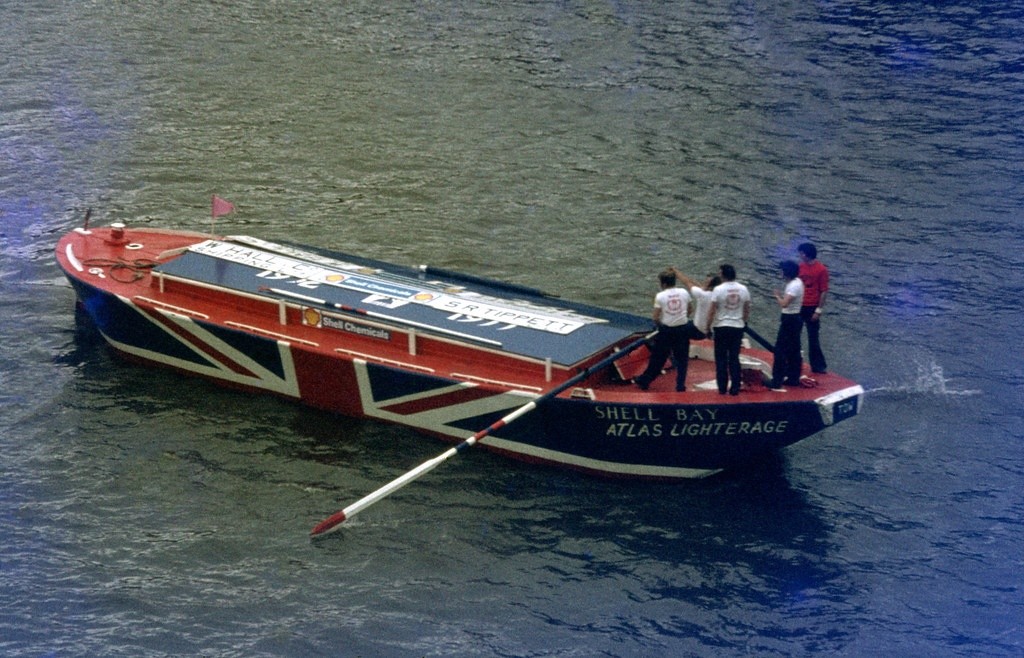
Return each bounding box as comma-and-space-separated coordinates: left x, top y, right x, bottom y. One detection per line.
763, 243, 829, 389
630, 263, 751, 396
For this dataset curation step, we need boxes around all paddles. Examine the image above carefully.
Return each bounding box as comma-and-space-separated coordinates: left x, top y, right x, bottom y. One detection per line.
306, 326, 660, 537
742, 323, 775, 352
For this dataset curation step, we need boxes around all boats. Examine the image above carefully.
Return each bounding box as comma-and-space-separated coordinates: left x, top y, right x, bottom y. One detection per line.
56, 220, 862, 486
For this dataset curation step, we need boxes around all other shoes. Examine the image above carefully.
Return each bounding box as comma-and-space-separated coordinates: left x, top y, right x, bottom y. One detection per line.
729, 387, 738, 395
761, 379, 780, 389
782, 377, 800, 386
676, 386, 685, 392
718, 388, 725, 394
812, 367, 827, 374
632, 376, 647, 390
